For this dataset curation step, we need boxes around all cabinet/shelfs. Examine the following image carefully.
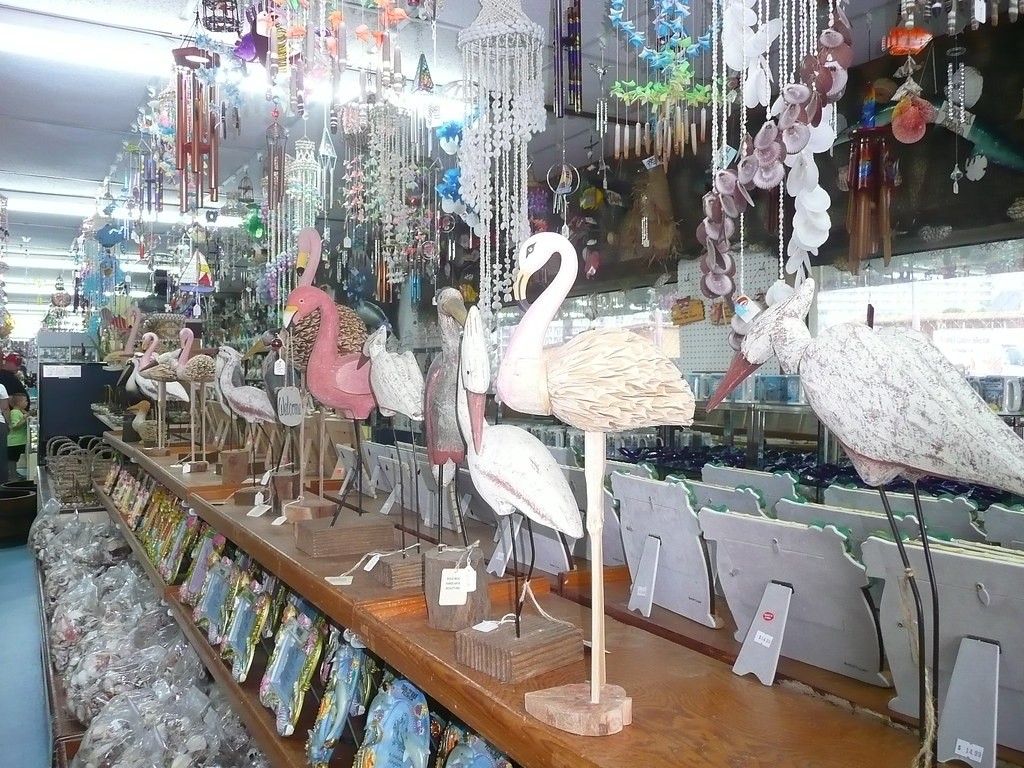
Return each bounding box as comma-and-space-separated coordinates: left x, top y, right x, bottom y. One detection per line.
41, 430, 1024, 768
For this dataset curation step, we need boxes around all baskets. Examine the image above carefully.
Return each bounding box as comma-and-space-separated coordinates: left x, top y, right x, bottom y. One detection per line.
44, 435, 117, 502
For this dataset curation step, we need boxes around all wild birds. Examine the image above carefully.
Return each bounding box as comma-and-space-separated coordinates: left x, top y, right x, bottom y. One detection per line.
496, 227, 698, 705
702, 278, 1024, 768
86, 227, 586, 633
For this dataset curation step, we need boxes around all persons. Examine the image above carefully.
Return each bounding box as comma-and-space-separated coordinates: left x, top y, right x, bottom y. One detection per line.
0, 350, 30, 414
7, 393, 33, 482
0, 383, 11, 484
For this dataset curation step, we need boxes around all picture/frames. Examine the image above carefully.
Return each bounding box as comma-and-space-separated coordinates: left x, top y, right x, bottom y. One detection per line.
204, 393, 1024, 768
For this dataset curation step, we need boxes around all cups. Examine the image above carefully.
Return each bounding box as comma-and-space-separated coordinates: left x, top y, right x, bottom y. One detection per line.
761, 375, 785, 404
520, 425, 584, 457
607, 427, 663, 458
708, 373, 731, 402
964, 375, 1024, 415
675, 429, 712, 450
683, 373, 711, 402
786, 375, 808, 405
735, 374, 761, 404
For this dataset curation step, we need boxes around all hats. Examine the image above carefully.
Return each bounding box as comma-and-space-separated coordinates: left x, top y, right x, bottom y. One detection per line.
2, 352, 22, 366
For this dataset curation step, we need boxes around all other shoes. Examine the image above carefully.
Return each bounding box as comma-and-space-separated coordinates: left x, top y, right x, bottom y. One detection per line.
7, 471, 25, 481
